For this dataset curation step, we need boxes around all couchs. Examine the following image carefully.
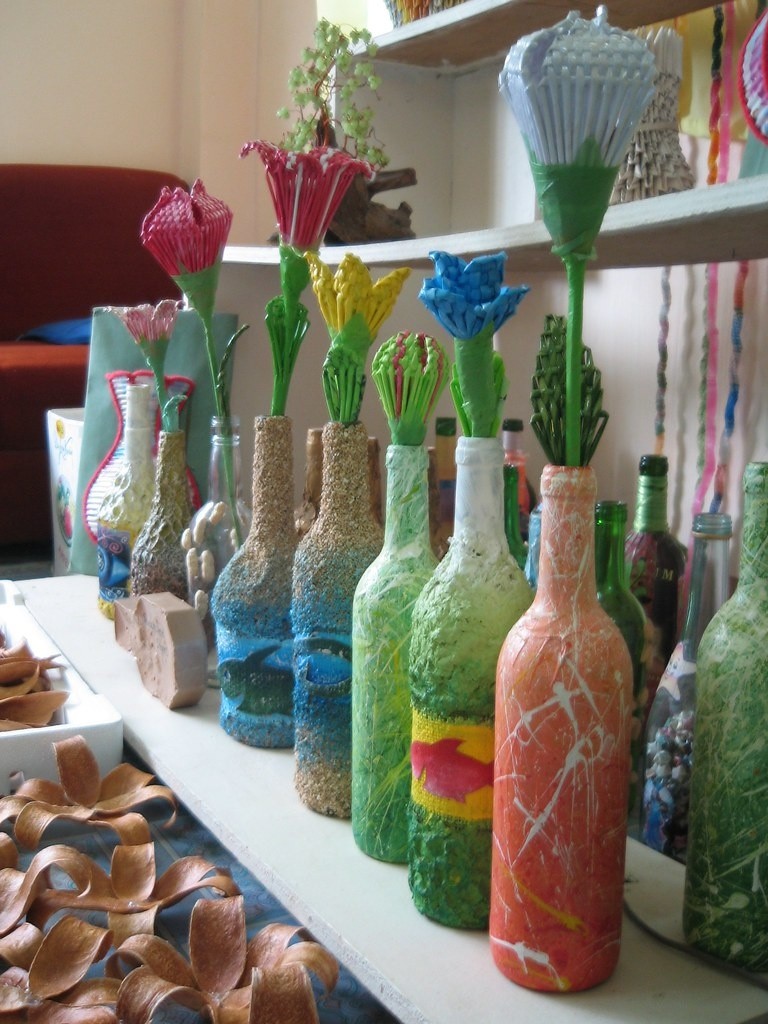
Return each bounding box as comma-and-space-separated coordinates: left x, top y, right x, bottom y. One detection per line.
0, 163, 192, 546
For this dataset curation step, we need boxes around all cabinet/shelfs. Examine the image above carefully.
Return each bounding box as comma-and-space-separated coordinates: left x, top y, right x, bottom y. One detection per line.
17, 0, 768, 1024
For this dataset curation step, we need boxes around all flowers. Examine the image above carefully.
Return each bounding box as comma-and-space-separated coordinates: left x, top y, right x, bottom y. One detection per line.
119, 3, 659, 468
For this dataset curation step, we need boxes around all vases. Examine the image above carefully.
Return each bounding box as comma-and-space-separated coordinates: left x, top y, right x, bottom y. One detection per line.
96, 382, 768, 994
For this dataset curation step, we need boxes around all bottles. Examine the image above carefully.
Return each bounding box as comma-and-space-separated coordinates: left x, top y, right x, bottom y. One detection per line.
683, 460, 768, 987
366, 432, 386, 525
591, 497, 647, 705
640, 513, 732, 863
521, 509, 542, 597
429, 415, 458, 562
490, 467, 636, 994
293, 428, 325, 540
292, 422, 385, 823
500, 418, 536, 570
96, 382, 157, 621
129, 427, 195, 605
180, 435, 251, 693
352, 443, 440, 866
408, 438, 532, 930
628, 455, 688, 668
210, 414, 300, 751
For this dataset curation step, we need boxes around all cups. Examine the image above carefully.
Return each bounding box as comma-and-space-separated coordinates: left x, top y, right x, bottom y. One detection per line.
45, 406, 86, 578
